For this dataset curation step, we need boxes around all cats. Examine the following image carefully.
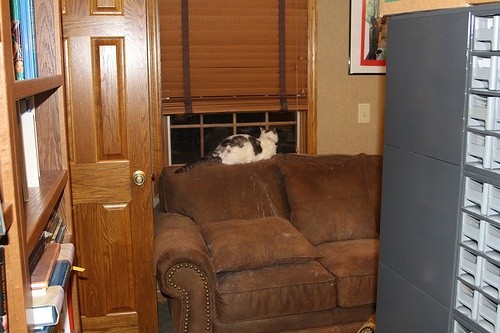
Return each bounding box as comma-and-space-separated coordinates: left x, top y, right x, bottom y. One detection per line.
174, 126, 279, 174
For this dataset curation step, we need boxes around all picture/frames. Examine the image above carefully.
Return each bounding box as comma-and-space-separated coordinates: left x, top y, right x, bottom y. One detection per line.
348, 0, 388, 75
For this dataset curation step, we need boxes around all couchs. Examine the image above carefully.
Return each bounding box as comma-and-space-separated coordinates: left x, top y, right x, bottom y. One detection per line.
154, 152, 383, 333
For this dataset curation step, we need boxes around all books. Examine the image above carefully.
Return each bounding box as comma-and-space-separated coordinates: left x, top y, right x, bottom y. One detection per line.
17, 96, 41, 203
26, 209, 75, 333
0, 201, 9, 333
8, 0, 37, 81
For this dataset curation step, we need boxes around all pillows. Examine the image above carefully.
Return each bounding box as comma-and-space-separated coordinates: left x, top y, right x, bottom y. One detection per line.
194, 215, 324, 275
347, 200, 347, 203
275, 152, 381, 246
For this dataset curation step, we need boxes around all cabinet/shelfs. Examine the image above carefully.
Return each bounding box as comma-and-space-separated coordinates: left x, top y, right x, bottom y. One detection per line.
446, 4, 500, 333
0, 0, 82, 333
